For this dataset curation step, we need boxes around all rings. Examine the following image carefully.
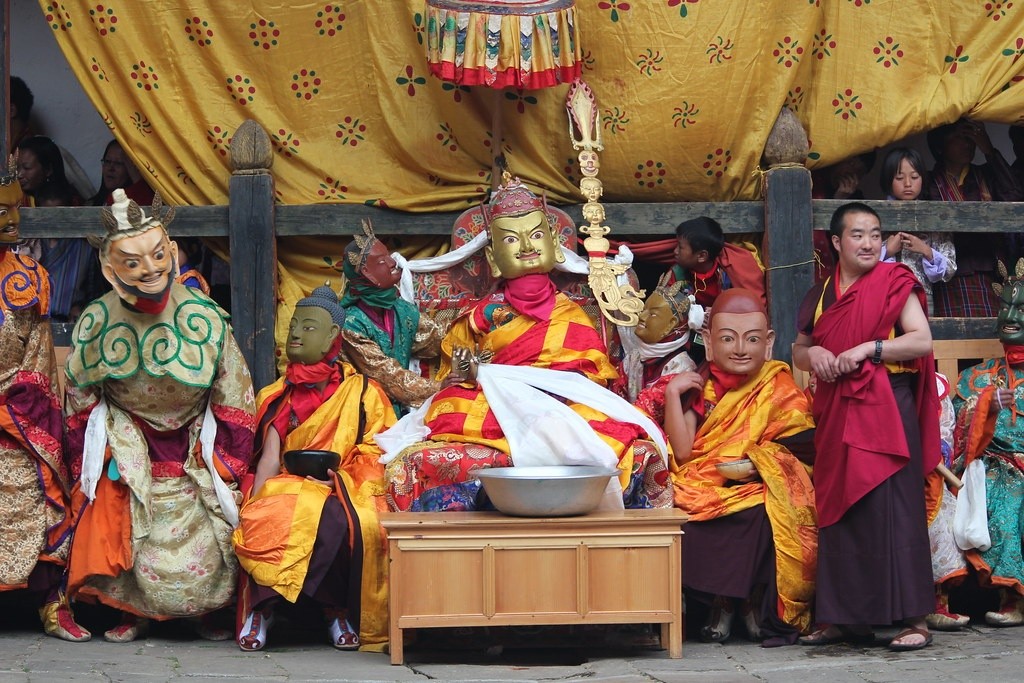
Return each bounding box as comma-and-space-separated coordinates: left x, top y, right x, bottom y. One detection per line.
973, 132, 977, 135
810, 383, 815, 387
974, 127, 982, 131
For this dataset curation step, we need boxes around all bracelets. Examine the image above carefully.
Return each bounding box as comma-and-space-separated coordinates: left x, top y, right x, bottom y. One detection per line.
872, 340, 883, 364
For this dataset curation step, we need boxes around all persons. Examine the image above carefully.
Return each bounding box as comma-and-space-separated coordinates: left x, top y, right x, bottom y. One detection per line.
336, 219, 504, 418
0, 147, 91, 642
793, 120, 1024, 650
374, 168, 697, 652
62, 188, 256, 642
656, 216, 769, 364
10, 74, 205, 321
231, 278, 398, 651
665, 288, 818, 642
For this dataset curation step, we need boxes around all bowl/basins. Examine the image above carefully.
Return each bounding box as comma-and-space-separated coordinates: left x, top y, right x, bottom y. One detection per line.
716, 459, 753, 480
283, 450, 342, 482
468, 466, 622, 517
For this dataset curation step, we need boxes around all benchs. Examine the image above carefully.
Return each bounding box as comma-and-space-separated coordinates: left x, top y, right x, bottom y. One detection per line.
792, 339, 1006, 399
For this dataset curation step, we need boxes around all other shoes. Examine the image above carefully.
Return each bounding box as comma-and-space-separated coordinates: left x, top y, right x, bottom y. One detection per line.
699, 597, 734, 641
740, 600, 766, 641
323, 609, 361, 649
986, 587, 1024, 625
238, 611, 267, 652
925, 591, 970, 629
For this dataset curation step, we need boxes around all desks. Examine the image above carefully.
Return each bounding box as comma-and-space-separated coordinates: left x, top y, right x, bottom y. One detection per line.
380, 507, 691, 666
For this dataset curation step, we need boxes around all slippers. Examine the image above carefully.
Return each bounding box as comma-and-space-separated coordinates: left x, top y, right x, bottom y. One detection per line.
889, 623, 932, 650
798, 623, 876, 644
